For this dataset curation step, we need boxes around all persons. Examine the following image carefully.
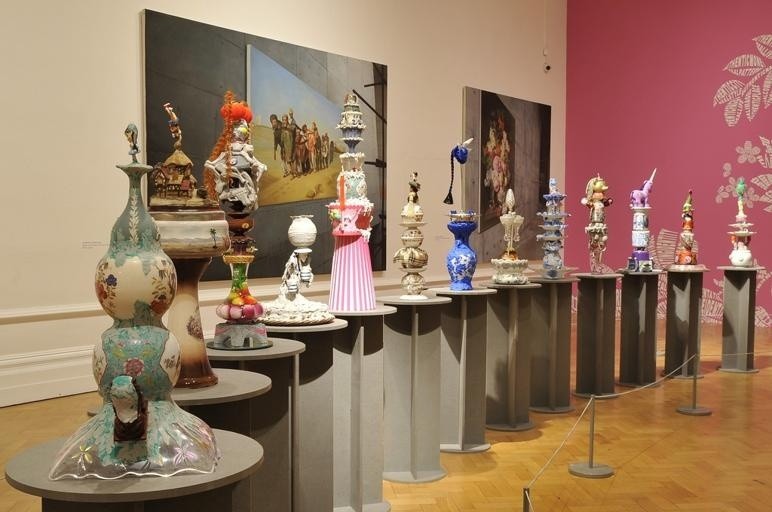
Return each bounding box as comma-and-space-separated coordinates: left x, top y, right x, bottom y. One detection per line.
401, 170, 425, 213
124, 122, 142, 164
164, 101, 183, 144
267, 110, 331, 178
